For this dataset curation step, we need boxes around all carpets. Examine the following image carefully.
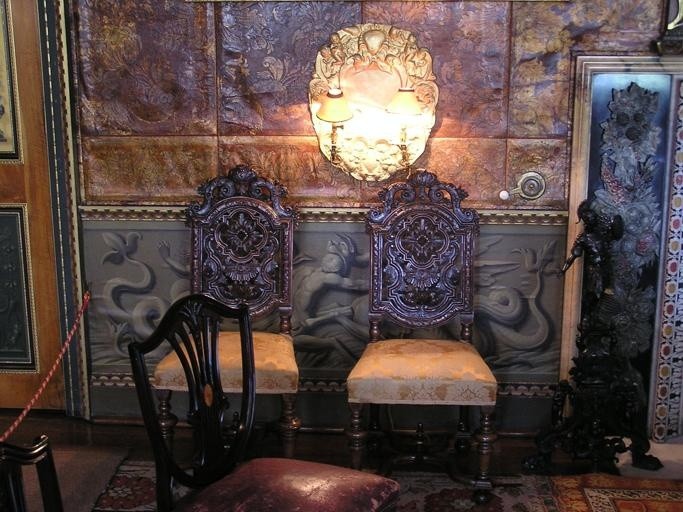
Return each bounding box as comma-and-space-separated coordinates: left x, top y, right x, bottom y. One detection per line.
88, 436, 682, 510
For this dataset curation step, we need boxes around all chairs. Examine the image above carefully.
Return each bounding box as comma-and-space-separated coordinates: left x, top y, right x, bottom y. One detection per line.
129, 294, 401, 508
0, 435, 63, 511
344, 171, 497, 505
149, 163, 298, 457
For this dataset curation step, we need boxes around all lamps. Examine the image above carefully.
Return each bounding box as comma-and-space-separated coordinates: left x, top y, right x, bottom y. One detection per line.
314, 87, 423, 181
